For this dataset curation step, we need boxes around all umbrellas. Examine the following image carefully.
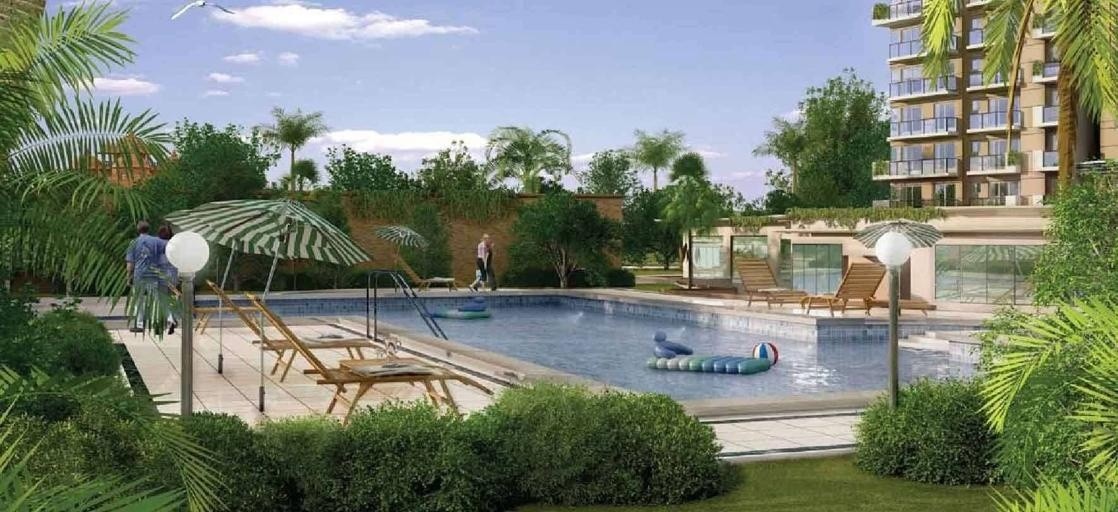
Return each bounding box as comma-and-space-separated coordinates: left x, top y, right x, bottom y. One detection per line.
372, 225, 432, 292
853, 218, 944, 318
187, 198, 375, 413
164, 209, 297, 374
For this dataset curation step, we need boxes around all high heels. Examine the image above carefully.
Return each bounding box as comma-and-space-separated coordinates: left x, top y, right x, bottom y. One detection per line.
168, 321, 176, 334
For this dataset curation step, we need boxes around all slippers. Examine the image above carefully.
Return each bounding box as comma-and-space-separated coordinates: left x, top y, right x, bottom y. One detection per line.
469, 285, 477, 293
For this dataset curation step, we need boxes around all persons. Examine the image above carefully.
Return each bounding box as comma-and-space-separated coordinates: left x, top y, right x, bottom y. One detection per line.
125, 220, 169, 335
154, 224, 178, 335
469, 234, 497, 294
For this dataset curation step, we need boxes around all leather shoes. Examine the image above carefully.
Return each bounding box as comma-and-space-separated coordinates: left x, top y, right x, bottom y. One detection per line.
130, 328, 143, 332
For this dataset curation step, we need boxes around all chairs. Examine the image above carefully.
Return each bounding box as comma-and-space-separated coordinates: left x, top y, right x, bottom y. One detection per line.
204, 279, 374, 383
166, 281, 260, 335
732, 254, 887, 317
243, 277, 494, 430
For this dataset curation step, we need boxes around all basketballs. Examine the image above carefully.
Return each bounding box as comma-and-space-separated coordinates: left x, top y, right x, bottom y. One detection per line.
753, 343, 779, 364
